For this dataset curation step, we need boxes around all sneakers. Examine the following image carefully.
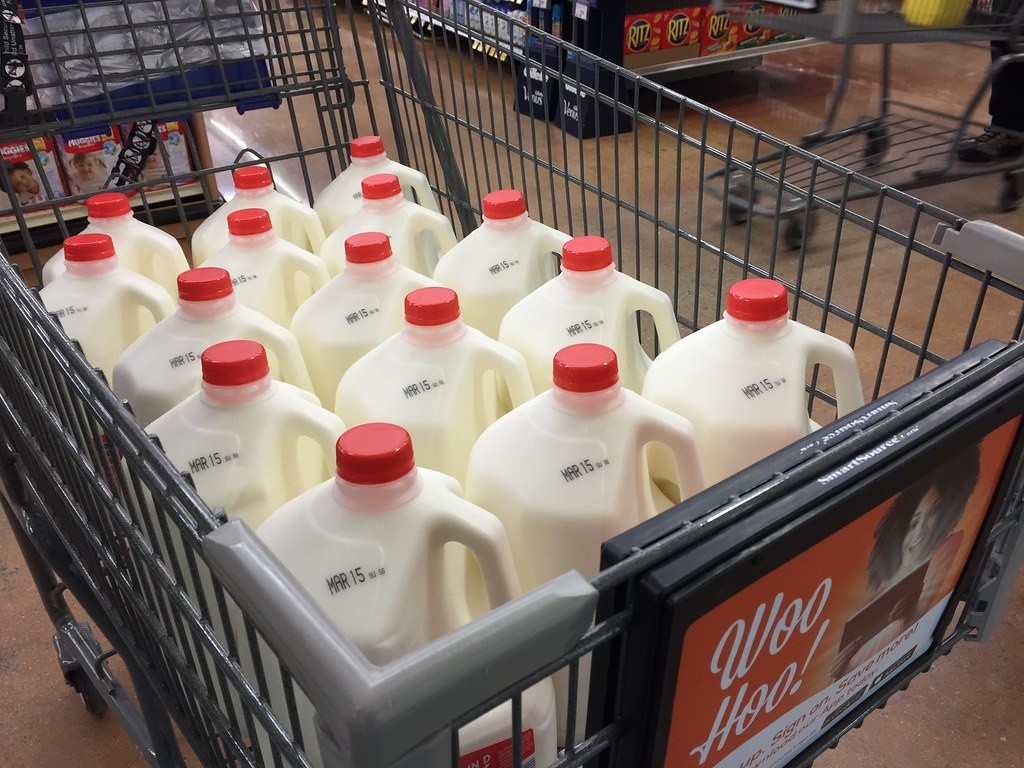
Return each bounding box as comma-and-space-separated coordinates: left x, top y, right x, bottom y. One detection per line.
958, 128, 1024, 163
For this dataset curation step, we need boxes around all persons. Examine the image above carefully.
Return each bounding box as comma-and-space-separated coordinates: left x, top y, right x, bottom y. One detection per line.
830, 440, 980, 683
0, 162, 47, 206
65, 153, 108, 195
957, 0, 1024, 161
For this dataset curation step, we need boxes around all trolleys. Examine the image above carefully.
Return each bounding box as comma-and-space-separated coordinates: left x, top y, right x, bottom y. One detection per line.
1, 0, 1024, 768
703, 0, 1023, 248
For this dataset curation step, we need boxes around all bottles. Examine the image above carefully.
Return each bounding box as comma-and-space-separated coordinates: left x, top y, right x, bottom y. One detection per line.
236, 423, 557, 768
288, 232, 449, 414
120, 338, 348, 748
497, 235, 683, 411
321, 175, 459, 282
641, 276, 865, 488
110, 267, 315, 428
463, 343, 706, 747
41, 191, 190, 307
312, 134, 440, 237
334, 288, 536, 493
36, 235, 178, 497
191, 167, 327, 266
433, 189, 574, 341
